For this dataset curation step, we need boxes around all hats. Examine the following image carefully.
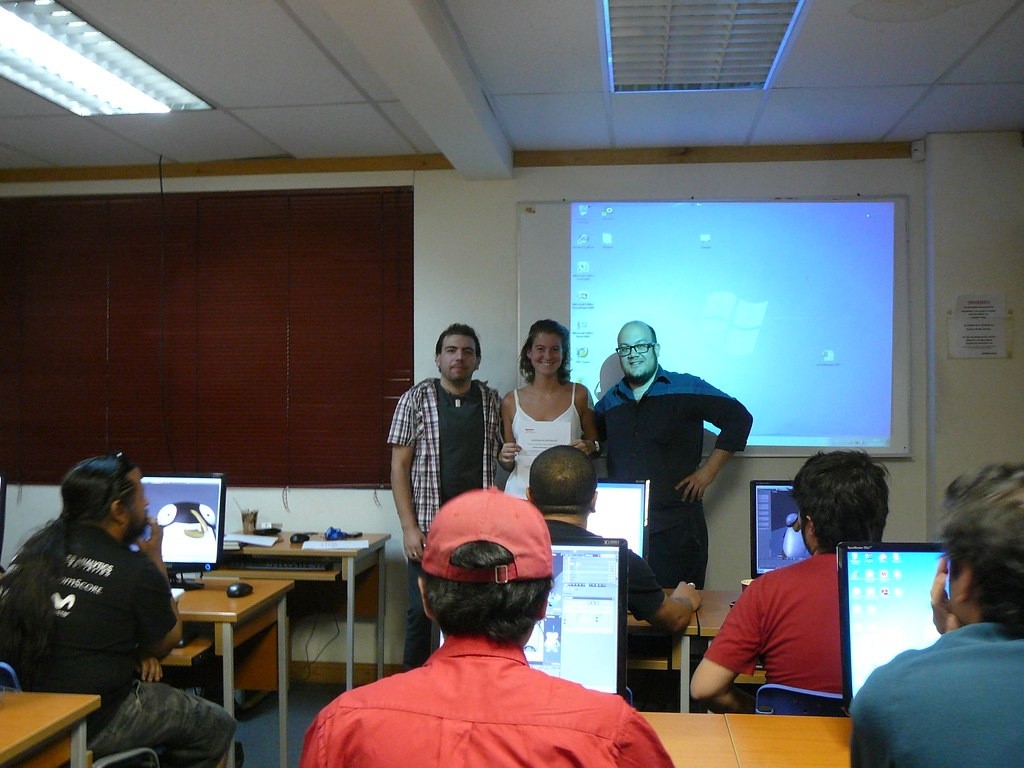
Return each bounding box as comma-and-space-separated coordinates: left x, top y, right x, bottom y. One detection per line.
421, 487, 553, 584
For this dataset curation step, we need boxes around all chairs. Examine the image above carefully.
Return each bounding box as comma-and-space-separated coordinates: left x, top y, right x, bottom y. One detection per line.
0, 662, 159, 768
755, 684, 849, 717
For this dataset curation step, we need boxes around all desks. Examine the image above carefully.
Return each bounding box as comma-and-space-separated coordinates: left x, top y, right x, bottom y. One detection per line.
695, 590, 766, 713
626, 589, 698, 713
159, 578, 295, 768
213, 530, 392, 692
0, 691, 102, 768
636, 712, 852, 768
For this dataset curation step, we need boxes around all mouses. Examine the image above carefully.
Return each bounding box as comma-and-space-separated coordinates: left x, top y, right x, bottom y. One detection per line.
291, 534, 308, 543
226, 583, 253, 597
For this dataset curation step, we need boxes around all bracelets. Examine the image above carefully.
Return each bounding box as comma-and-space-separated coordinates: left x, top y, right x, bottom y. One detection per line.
593, 441, 599, 452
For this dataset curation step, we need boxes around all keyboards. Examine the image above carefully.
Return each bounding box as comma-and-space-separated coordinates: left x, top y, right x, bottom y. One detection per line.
228, 556, 334, 571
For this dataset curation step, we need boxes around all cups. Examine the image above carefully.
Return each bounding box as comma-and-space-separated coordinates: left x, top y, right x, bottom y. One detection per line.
242, 510, 257, 534
741, 579, 754, 592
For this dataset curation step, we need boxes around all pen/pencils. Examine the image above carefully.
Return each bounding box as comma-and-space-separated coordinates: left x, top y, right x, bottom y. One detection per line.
304, 533, 318, 535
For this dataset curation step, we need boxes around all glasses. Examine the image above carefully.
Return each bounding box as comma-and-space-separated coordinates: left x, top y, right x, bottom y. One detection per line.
108, 451, 128, 494
616, 344, 654, 356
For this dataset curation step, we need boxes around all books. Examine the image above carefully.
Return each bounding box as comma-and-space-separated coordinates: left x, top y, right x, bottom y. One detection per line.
302, 539, 369, 549
224, 534, 278, 550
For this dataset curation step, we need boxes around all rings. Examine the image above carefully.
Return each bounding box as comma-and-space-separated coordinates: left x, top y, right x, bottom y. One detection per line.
412, 552, 418, 556
422, 544, 426, 547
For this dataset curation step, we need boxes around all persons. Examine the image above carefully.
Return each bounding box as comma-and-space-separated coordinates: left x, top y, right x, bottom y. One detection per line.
690, 450, 891, 712
848, 461, 1024, 768
387, 324, 502, 673
301, 485, 677, 768
0, 455, 245, 768
525, 445, 701, 633
497, 318, 599, 500
590, 320, 754, 590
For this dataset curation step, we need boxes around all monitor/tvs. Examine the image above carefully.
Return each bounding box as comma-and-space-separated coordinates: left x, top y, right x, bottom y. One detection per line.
835, 540, 956, 715
430, 537, 627, 704
749, 479, 813, 579
126, 471, 226, 591
586, 478, 650, 565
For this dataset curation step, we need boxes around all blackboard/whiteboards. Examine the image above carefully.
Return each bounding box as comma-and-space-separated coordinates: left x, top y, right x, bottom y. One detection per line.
518, 199, 910, 458
